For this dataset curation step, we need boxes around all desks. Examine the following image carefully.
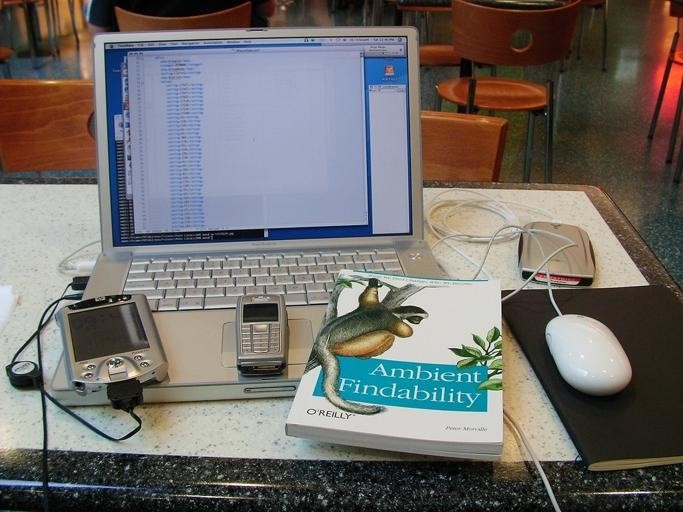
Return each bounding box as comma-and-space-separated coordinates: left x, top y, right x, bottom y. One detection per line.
1, 176, 683, 512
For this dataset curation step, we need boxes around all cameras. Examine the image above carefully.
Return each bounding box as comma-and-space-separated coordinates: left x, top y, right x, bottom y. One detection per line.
56, 291, 169, 397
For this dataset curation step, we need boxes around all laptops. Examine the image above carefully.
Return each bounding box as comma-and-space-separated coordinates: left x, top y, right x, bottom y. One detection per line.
51, 24, 449, 405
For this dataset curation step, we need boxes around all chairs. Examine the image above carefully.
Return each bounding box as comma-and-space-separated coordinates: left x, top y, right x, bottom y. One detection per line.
1, 0, 683, 182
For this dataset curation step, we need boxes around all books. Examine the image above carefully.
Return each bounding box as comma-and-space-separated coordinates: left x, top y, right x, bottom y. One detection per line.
281, 268, 507, 463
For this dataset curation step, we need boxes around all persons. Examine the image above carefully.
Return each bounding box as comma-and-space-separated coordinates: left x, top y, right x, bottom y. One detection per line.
82, 1, 277, 36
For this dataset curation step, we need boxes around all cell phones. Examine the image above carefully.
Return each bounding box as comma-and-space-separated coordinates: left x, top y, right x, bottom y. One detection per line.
236, 293, 289, 376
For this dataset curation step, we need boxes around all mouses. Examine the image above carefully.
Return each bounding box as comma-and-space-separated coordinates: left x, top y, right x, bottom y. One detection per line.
544, 314, 633, 397
